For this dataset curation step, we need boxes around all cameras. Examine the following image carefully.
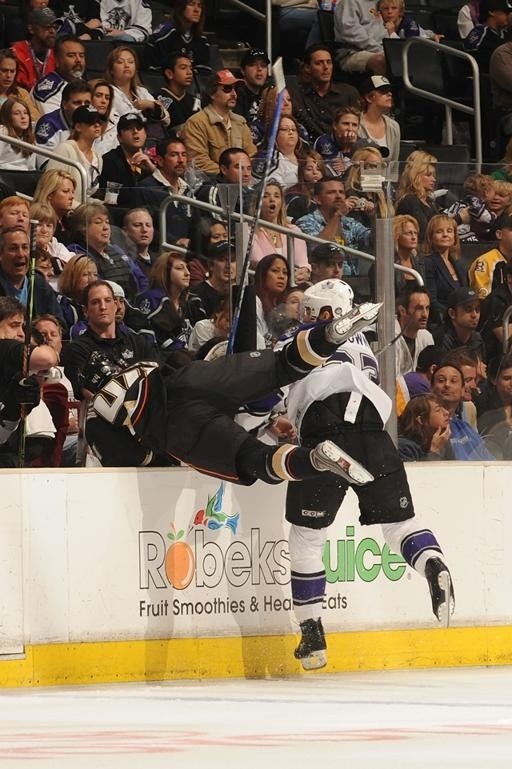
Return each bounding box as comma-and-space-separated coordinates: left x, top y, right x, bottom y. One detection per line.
352, 199, 366, 207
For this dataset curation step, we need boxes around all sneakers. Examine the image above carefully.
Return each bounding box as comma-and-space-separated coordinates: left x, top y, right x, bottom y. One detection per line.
324, 302, 380, 346
293, 616, 328, 660
424, 556, 456, 624
308, 441, 370, 486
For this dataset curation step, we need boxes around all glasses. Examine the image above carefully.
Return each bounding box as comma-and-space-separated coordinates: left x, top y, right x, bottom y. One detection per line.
217, 85, 243, 95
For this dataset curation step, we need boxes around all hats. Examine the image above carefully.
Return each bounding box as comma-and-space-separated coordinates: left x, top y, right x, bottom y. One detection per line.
73, 104, 108, 125
240, 47, 271, 68
360, 73, 395, 98
211, 240, 236, 259
24, 5, 67, 30
101, 279, 126, 300
480, 1, 512, 14
446, 287, 485, 311
116, 113, 146, 133
205, 68, 245, 92
350, 138, 390, 158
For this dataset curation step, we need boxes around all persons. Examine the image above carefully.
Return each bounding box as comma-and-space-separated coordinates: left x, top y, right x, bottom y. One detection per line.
264, 278, 456, 658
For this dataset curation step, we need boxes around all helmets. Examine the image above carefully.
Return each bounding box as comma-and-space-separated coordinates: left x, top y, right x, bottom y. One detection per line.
75, 348, 130, 395
302, 277, 356, 326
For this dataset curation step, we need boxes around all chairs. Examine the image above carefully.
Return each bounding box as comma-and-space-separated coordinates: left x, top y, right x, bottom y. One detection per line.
105, 206, 130, 227
341, 271, 374, 297
0, 168, 45, 201
78, 37, 298, 104
453, 241, 497, 277
249, 0, 470, 202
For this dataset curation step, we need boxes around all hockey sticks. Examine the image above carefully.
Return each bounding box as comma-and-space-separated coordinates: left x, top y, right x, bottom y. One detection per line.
227, 54, 287, 354
